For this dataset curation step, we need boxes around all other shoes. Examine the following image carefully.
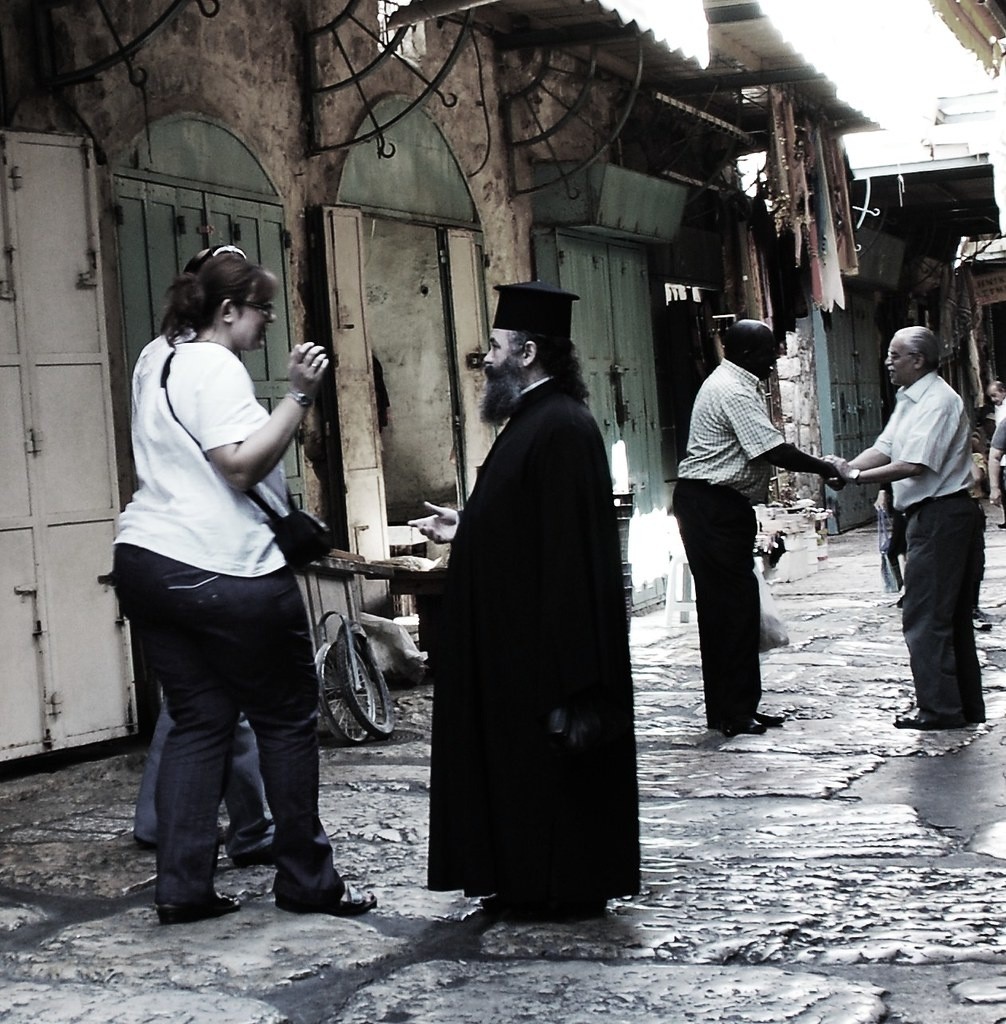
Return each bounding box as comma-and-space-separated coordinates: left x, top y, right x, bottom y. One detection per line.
895, 706, 986, 730
231, 842, 279, 869
132, 832, 158, 851
479, 891, 611, 925
895, 585, 907, 607
707, 711, 786, 734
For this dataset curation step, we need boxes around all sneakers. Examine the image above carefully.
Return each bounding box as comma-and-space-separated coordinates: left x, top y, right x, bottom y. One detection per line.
971, 610, 993, 631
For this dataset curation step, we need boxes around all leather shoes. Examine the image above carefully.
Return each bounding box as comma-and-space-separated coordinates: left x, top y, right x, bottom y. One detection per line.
274, 881, 377, 917
157, 888, 243, 924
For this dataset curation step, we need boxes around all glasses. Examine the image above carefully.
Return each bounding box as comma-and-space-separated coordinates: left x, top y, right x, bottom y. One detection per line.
887, 352, 924, 361
233, 300, 275, 314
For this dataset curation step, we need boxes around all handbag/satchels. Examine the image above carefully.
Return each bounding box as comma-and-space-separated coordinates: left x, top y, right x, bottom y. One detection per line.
271, 508, 335, 572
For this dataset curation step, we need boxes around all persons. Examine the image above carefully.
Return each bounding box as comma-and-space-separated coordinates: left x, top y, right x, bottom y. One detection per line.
111, 243, 379, 926
669, 318, 847, 735
818, 325, 994, 731
388, 281, 643, 925
984, 380, 1006, 529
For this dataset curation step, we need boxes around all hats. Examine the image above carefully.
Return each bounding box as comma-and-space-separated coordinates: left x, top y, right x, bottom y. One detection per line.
493, 277, 582, 339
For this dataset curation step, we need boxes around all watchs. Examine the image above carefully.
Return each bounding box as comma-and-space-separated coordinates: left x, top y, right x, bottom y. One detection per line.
285, 391, 313, 407
848, 469, 861, 486
